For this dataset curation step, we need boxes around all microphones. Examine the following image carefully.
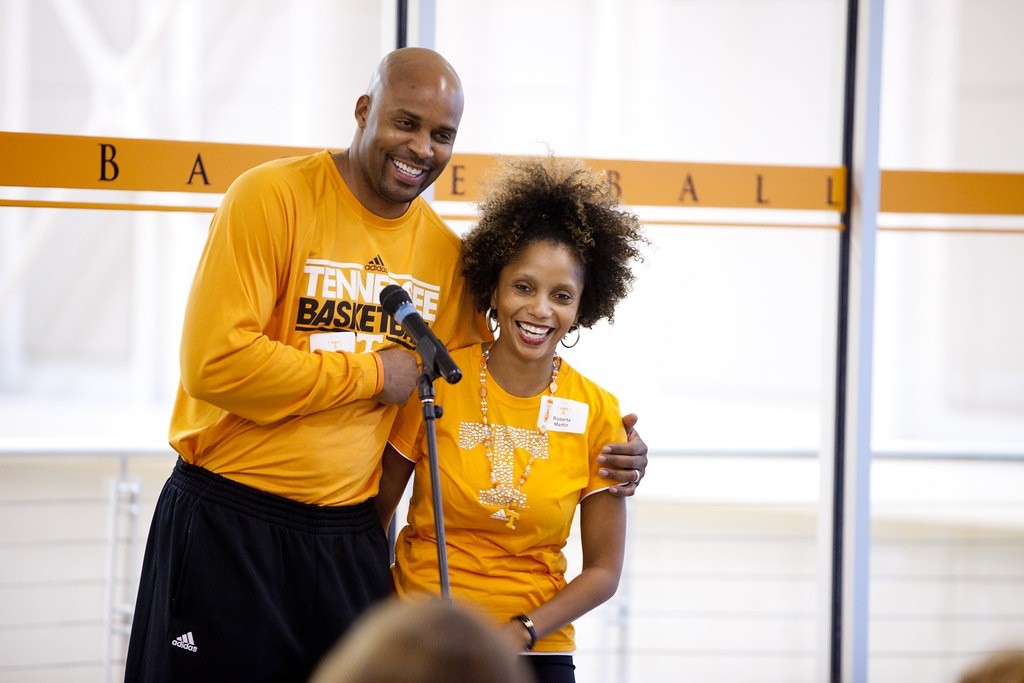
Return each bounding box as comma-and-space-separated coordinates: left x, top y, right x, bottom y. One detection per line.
378, 284, 462, 383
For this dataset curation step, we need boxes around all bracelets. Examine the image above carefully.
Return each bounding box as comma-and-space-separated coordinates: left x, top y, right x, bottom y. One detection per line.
511, 613, 537, 650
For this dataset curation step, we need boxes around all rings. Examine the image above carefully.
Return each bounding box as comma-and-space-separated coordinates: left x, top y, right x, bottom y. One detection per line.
634, 470, 640, 483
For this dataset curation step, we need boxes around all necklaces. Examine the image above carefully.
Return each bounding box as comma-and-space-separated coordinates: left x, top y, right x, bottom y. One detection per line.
478, 340, 561, 499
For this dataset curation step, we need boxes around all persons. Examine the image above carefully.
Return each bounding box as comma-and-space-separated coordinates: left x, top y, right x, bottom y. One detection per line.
375, 155, 628, 683
124, 45, 648, 683
314, 591, 531, 683
